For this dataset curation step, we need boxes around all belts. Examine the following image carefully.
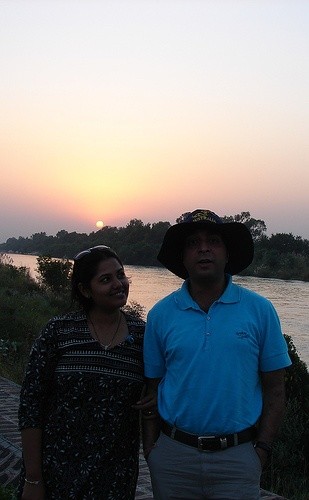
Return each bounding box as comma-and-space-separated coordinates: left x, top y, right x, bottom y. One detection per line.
160, 423, 251, 453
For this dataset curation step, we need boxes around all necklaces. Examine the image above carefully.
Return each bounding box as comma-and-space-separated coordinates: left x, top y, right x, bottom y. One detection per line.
90, 311, 122, 352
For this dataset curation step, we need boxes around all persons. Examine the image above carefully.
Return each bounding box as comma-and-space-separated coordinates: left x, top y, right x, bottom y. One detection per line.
142, 209, 292, 500
16, 245, 158, 500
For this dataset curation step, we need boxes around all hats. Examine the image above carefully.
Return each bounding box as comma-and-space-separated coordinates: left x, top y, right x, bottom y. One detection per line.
157, 209, 254, 280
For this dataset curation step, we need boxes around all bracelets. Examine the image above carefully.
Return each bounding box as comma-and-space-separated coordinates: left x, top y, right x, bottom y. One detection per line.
25, 479, 43, 485
144, 448, 151, 456
258, 434, 274, 457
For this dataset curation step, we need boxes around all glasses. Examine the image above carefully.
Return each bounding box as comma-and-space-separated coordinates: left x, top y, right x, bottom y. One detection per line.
73, 245, 111, 273
